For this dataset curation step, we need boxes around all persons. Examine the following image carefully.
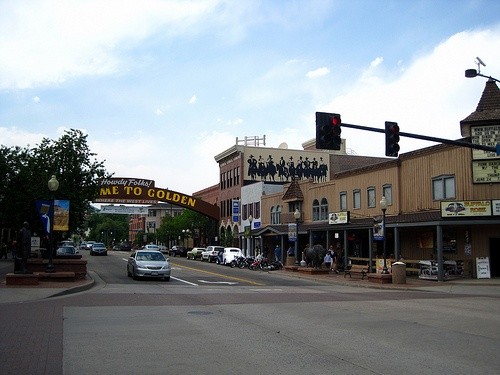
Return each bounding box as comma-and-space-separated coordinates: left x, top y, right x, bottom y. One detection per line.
0, 238, 17, 260
324, 244, 345, 272
19, 221, 31, 248
274, 245, 281, 261
287, 245, 295, 255
247, 153, 328, 177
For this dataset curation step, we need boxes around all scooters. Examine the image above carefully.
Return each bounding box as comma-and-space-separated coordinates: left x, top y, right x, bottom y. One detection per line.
230, 255, 283, 270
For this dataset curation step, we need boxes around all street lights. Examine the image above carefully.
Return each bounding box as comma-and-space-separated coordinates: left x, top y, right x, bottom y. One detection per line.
182, 229, 185, 246
186, 229, 190, 249
45, 174, 60, 273
249, 215, 253, 258
293, 209, 301, 265
379, 197, 390, 279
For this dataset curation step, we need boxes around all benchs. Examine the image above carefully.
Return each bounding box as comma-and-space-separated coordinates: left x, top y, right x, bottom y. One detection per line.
283, 265, 301, 272
344, 270, 368, 280
6, 272, 75, 287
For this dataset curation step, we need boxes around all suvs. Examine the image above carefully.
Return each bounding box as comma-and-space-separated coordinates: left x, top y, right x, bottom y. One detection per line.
201, 246, 224, 262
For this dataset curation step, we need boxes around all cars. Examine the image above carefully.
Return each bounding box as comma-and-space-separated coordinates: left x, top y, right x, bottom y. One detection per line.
168, 245, 186, 257
127, 249, 171, 281
90, 243, 108, 255
145, 244, 159, 250
113, 246, 121, 250
61, 241, 76, 247
187, 247, 205, 260
57, 245, 79, 254
216, 247, 246, 266
80, 241, 96, 250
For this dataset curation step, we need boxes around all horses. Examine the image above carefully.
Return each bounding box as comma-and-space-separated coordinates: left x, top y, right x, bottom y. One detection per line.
244, 161, 328, 184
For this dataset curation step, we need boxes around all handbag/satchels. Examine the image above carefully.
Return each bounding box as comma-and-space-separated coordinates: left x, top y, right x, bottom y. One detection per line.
333, 262, 337, 270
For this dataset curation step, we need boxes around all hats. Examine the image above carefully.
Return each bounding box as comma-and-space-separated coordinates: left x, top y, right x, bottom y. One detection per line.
330, 245, 333, 248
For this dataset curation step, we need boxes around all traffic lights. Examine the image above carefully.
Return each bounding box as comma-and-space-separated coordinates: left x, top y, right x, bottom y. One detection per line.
315, 111, 341, 150
384, 121, 400, 158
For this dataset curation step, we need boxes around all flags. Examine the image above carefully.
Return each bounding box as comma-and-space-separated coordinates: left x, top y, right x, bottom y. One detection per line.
39, 199, 54, 234
53, 200, 70, 231
